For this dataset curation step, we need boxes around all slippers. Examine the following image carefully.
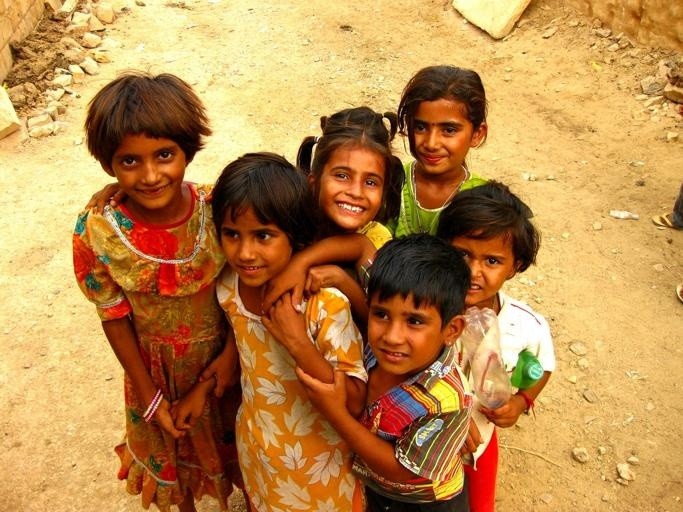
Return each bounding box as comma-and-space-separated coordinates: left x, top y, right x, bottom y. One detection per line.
652, 212, 674, 229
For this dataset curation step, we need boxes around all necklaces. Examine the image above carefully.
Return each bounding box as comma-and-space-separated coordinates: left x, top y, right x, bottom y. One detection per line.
413, 162, 468, 236
489, 295, 496, 317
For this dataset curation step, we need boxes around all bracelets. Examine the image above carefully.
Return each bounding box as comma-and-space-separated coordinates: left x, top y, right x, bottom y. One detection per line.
515, 390, 536, 419
141, 387, 160, 420
144, 393, 163, 422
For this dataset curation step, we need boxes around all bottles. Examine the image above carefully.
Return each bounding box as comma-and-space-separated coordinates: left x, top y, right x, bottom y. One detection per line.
459, 306, 512, 410
508, 350, 543, 391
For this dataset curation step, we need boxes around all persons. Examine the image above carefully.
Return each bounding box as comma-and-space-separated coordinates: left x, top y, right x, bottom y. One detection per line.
316, 106, 403, 143
294, 130, 407, 325
379, 63, 487, 240
258, 230, 468, 512
430, 178, 556, 512
165, 149, 368, 512
70, 69, 250, 512
650, 178, 682, 307
